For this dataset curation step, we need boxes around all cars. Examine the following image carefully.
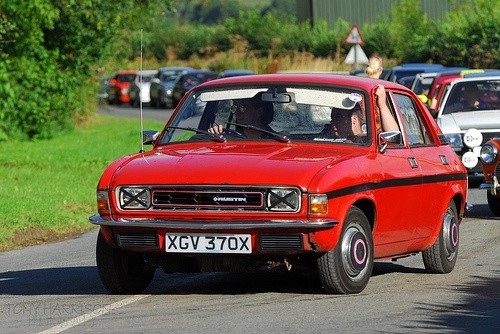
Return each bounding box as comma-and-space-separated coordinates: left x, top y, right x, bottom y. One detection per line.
107, 71, 138, 105
88, 71, 469, 295
440, 76, 491, 81
175, 72, 256, 110
350, 63, 500, 120
437, 77, 500, 174
134, 70, 159, 108
481, 138, 500, 217
150, 67, 195, 109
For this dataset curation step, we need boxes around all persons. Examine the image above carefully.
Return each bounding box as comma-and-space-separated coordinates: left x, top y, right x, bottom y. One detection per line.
445, 83, 491, 115
330, 84, 400, 144
366, 52, 382, 82
207, 93, 273, 139
421, 85, 431, 96
162, 73, 212, 87
